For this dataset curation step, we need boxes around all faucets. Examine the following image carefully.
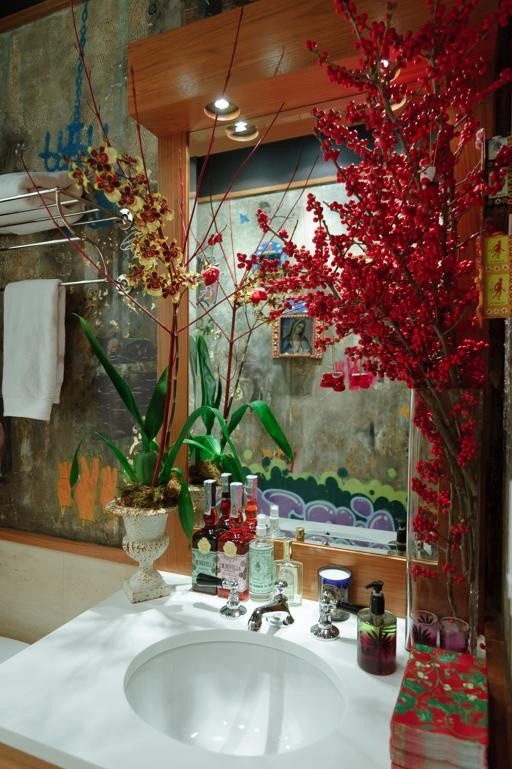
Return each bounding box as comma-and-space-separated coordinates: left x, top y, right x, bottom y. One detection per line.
248, 578, 295, 632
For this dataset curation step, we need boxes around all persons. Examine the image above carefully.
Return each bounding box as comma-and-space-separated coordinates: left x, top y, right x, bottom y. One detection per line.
285, 333, 305, 355
282, 317, 312, 355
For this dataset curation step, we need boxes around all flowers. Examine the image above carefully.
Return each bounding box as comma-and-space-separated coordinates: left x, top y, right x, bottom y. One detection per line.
18, 0, 320, 488
233, 3, 511, 650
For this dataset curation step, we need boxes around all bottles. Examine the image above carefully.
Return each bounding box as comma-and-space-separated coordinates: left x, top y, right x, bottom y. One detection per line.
217, 471, 306, 544
190, 479, 303, 607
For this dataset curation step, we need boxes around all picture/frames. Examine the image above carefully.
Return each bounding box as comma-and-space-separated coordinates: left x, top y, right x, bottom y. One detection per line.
270, 311, 322, 359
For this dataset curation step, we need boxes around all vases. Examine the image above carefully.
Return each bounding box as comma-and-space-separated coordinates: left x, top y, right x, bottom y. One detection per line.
404, 389, 482, 657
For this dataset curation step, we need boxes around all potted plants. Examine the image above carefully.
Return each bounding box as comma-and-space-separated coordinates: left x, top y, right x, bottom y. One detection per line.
67, 310, 249, 605
188, 332, 296, 526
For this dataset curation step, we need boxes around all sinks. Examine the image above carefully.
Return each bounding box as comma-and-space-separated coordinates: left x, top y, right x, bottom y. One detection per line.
0, 571, 412, 769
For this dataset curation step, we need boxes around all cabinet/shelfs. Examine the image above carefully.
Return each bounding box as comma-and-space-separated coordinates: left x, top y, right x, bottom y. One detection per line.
0, 186, 135, 286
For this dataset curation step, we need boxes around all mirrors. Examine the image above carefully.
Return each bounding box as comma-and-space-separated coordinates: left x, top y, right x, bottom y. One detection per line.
125, 0, 494, 638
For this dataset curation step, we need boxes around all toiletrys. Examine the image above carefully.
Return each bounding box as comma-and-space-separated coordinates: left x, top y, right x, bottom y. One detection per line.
268, 505, 285, 539
248, 513, 275, 602
273, 537, 305, 607
294, 526, 306, 547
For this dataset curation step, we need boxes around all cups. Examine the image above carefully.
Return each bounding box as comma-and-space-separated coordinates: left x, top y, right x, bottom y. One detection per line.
317, 565, 351, 623
404, 612, 470, 653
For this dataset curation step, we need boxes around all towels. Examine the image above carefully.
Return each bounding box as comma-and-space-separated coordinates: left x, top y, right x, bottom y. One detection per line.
0, 167, 85, 422
